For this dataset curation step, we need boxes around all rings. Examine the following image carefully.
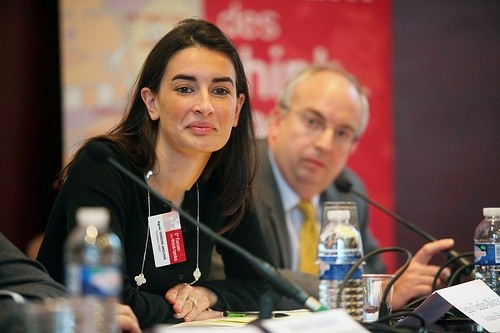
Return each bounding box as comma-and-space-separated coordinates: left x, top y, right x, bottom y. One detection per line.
186, 297, 197, 307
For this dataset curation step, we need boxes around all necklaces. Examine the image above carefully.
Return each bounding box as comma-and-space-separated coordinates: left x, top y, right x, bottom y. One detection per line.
135, 180, 201, 292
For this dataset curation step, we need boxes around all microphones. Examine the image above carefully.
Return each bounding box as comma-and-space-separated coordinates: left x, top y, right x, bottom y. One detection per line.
332, 176, 477, 280
84, 140, 328, 314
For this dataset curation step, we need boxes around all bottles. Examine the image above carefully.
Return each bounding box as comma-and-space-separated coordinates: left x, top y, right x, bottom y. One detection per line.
474, 207, 500, 329
318, 205, 365, 322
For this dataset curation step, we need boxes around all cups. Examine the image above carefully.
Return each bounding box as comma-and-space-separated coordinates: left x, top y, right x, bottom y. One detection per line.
362, 274, 394, 320
22, 296, 121, 333
63, 210, 123, 332
317, 201, 367, 265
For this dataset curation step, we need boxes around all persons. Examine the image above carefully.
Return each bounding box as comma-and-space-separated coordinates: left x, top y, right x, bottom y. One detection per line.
36, 19, 286, 328
209, 65, 455, 311
0, 229, 142, 333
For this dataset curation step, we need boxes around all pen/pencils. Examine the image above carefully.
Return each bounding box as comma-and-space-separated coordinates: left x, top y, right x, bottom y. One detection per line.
223, 311, 289, 318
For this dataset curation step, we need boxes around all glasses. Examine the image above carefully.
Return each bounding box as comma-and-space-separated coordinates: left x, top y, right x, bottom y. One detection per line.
281, 105, 361, 148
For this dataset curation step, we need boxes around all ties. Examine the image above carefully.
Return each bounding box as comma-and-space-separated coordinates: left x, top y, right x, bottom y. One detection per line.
298, 199, 320, 276
